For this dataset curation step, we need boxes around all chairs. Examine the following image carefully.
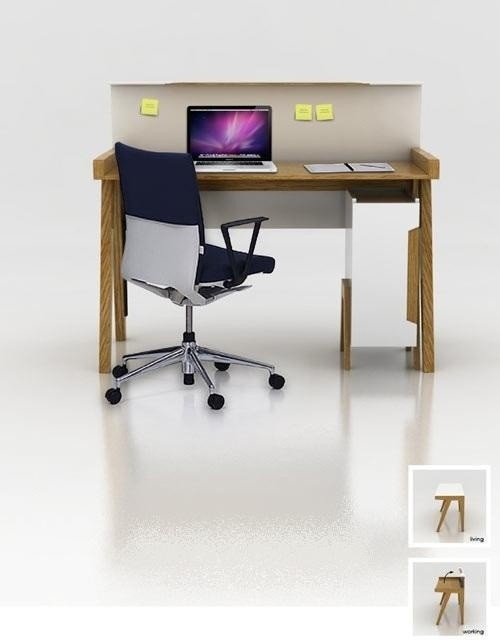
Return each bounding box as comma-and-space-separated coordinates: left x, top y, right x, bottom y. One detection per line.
104, 141, 287, 410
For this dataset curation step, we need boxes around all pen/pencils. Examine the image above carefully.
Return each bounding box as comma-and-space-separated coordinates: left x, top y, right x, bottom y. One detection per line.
344, 162, 354, 171
361, 164, 385, 168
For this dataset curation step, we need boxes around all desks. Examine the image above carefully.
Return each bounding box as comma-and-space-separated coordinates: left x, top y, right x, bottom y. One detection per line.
434, 577, 467, 625
434, 482, 466, 533
91, 146, 443, 373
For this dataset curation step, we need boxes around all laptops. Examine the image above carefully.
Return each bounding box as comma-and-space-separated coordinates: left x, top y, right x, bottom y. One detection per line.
186, 105, 278, 174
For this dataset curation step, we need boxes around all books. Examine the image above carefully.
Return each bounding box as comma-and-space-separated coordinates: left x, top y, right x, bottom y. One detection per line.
303, 163, 395, 174
